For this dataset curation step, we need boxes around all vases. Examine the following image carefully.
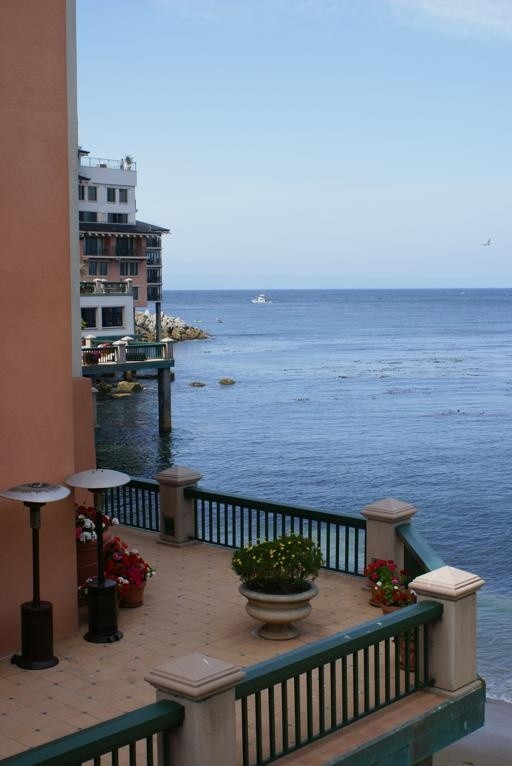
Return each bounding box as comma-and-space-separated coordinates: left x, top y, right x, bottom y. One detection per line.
368, 583, 395, 617
238, 577, 321, 641
77, 536, 108, 565
116, 580, 146, 611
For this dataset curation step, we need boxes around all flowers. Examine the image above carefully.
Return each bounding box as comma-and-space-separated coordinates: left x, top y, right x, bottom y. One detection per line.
85, 537, 152, 605
366, 556, 418, 609
231, 534, 324, 595
75, 502, 119, 544
